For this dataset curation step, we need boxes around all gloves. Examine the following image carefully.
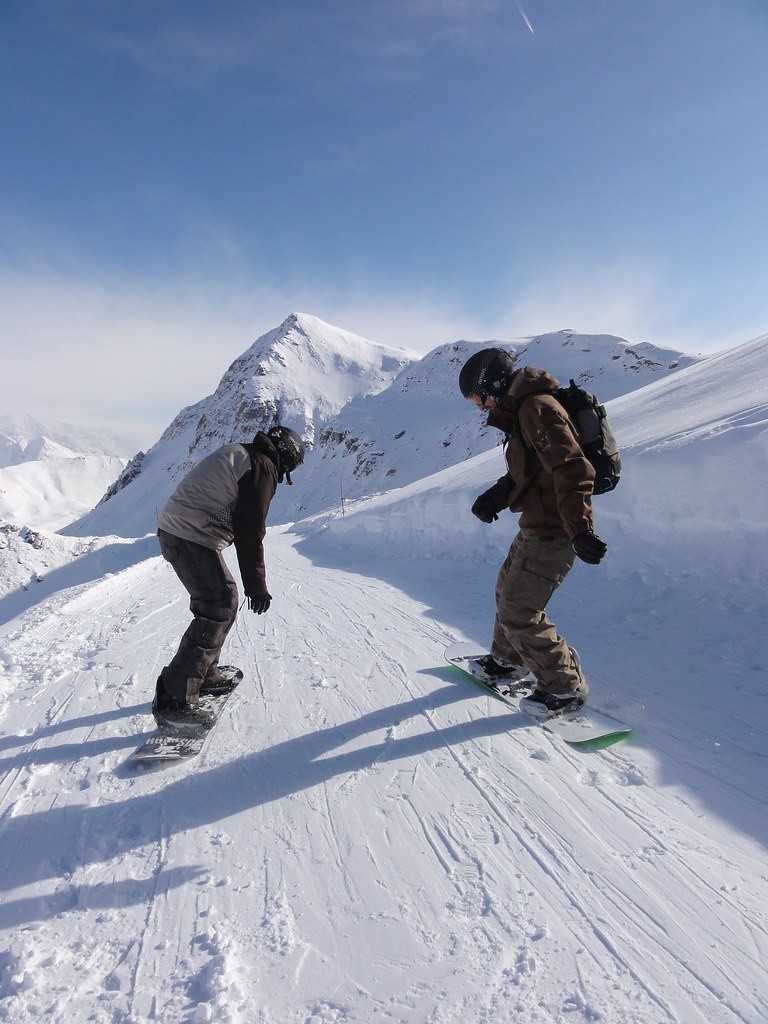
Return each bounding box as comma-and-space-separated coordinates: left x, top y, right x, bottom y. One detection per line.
471, 493, 503, 523
571, 530, 607, 564
249, 591, 273, 615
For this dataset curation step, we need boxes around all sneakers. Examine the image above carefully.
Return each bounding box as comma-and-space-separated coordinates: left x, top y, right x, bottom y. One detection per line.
197, 665, 233, 698
519, 685, 587, 720
150, 676, 218, 733
468, 651, 531, 685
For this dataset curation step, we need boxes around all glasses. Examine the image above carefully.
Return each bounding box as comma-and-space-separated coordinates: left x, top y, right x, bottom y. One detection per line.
466, 389, 485, 408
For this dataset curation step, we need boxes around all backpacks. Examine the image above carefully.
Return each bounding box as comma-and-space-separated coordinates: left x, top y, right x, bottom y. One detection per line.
518, 378, 621, 494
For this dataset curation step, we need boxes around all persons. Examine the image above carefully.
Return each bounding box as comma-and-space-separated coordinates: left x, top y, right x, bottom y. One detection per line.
152, 425, 305, 736
460, 349, 607, 715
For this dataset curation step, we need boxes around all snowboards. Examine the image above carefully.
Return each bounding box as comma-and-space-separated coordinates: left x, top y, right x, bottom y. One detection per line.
443, 640, 635, 745
125, 665, 246, 763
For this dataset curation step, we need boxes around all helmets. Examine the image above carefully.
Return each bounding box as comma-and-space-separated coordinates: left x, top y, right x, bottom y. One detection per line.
459, 347, 514, 400
268, 426, 305, 472
